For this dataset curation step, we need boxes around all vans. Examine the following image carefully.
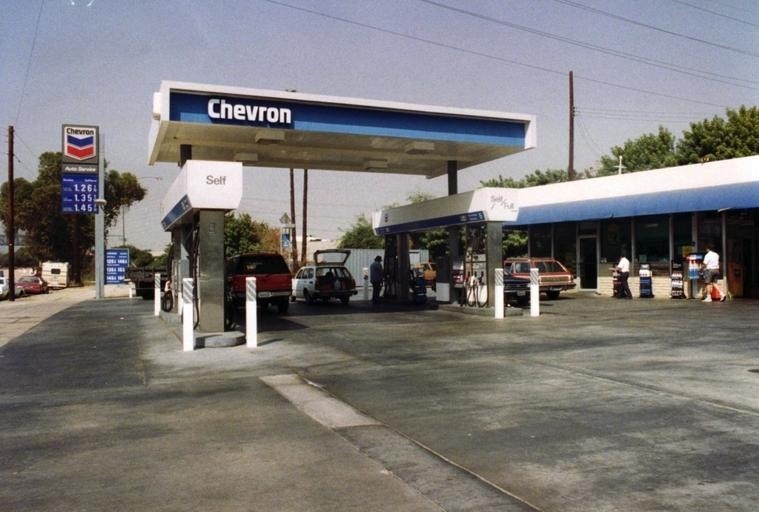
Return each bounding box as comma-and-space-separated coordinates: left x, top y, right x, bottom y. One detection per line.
41, 262, 70, 288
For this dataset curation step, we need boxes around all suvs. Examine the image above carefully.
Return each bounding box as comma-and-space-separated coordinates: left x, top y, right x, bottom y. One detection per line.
226, 250, 293, 313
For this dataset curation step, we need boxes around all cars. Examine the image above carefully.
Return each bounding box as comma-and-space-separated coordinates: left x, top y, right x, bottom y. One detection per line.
291, 250, 358, 304
0, 275, 50, 299
504, 259, 575, 305
416, 262, 437, 289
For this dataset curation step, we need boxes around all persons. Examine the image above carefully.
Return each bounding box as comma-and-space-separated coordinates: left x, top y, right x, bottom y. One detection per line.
701, 243, 727, 303
369, 255, 384, 305
609, 254, 633, 300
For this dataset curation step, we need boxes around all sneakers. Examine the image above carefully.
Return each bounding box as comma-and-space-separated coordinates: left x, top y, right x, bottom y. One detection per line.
719, 294, 726, 302
701, 298, 713, 302
